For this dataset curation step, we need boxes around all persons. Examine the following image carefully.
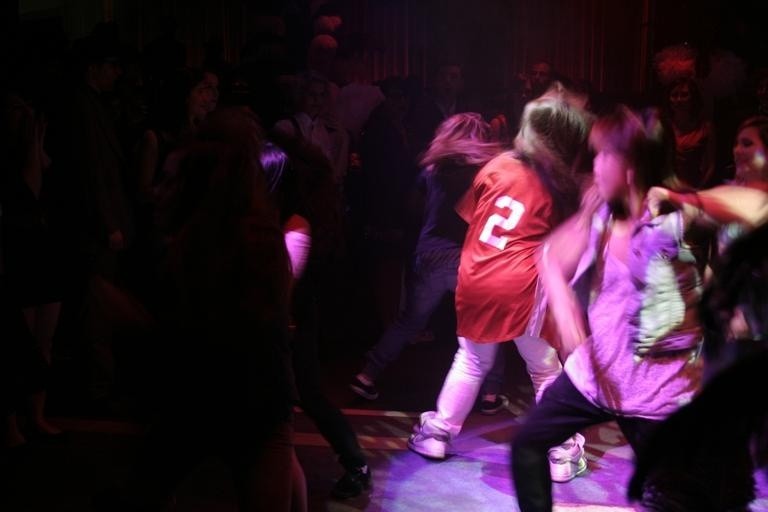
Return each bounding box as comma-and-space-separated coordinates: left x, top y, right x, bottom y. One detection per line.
2, 35, 768, 511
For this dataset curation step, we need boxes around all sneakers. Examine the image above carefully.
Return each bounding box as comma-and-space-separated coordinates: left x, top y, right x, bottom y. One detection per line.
549, 456, 588, 482
350, 374, 378, 400
334, 462, 372, 498
408, 433, 448, 459
481, 395, 509, 415
